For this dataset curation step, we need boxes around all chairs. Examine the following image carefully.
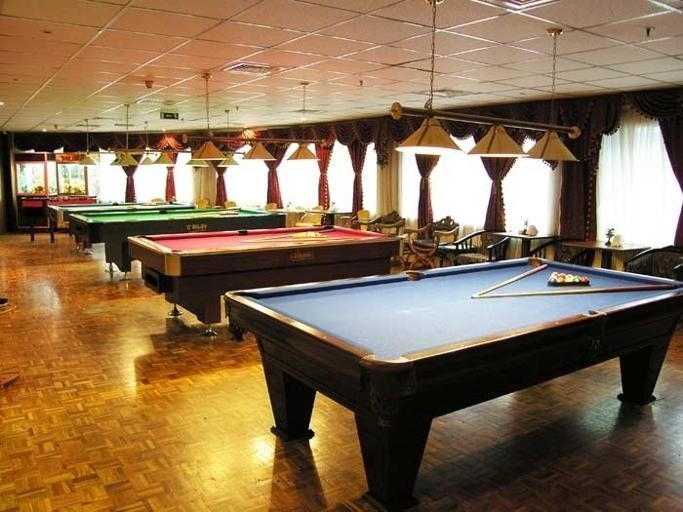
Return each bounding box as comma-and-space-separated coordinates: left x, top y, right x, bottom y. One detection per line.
141, 187, 683, 286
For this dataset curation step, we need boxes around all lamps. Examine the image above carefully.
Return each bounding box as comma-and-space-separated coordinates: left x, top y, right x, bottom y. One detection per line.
390, 1, 590, 164
75, 72, 323, 167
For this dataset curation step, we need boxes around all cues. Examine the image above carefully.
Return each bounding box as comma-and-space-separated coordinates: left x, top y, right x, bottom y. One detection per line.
470, 284, 675, 298
240, 229, 357, 243
470, 262, 548, 297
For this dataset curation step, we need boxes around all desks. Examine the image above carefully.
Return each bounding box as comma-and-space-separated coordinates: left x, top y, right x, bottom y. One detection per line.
59, 200, 226, 257
86, 209, 287, 282
225, 257, 682, 511
126, 224, 399, 338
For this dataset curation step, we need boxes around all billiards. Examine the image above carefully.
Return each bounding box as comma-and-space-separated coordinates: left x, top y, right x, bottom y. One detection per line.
548, 271, 588, 283
293, 231, 328, 239
